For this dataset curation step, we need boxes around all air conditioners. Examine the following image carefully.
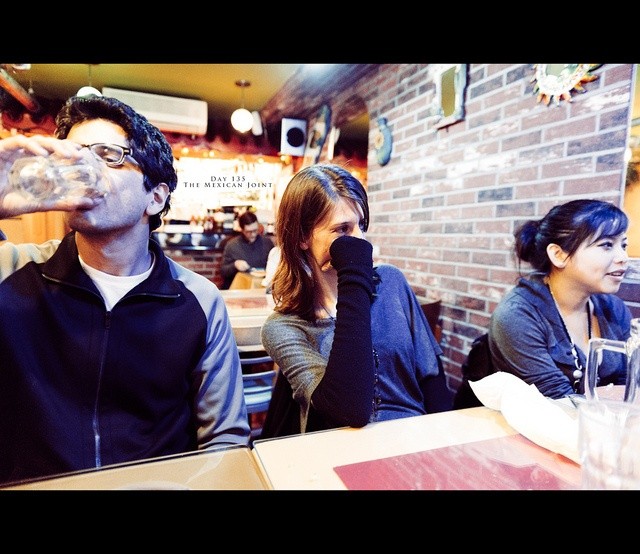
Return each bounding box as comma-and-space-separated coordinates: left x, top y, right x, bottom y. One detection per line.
101, 85, 209, 136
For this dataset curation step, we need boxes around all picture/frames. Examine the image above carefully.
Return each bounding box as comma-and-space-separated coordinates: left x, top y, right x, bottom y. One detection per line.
430, 62, 468, 129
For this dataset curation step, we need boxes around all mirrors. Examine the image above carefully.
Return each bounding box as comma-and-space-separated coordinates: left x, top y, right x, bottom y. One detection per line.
618, 62, 640, 259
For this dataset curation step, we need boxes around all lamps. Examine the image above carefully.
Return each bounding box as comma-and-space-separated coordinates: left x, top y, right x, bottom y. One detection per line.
230, 107, 254, 133
76, 85, 103, 97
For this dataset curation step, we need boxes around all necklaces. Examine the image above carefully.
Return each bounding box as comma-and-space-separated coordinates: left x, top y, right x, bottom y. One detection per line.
317, 296, 383, 417
545, 277, 592, 394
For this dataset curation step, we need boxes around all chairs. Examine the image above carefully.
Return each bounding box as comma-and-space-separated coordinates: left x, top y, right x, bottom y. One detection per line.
422, 295, 442, 338
225, 324, 281, 436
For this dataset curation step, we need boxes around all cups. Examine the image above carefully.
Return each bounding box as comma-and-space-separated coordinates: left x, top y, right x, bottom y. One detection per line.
7, 153, 112, 201
585, 318, 639, 405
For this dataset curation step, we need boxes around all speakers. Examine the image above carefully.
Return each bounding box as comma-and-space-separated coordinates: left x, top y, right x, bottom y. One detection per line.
277, 114, 310, 159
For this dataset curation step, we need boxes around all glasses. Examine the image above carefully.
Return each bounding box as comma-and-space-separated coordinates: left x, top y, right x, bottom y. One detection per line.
82, 143, 159, 185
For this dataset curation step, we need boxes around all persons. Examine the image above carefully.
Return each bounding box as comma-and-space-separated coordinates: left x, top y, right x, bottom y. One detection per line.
1, 94, 252, 486
488, 199, 634, 399
219, 210, 274, 290
261, 163, 453, 433
